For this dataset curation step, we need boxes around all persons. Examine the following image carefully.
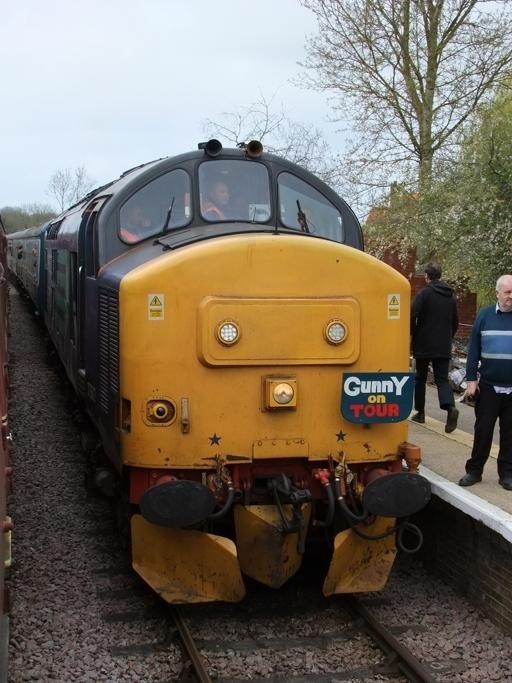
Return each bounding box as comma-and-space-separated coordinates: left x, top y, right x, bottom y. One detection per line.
200, 180, 232, 221
458, 277, 512, 491
411, 263, 460, 433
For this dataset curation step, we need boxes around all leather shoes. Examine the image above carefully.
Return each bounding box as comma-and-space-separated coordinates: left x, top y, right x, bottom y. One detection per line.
459, 472, 482, 485
445, 407, 459, 433
411, 411, 425, 423
498, 476, 512, 490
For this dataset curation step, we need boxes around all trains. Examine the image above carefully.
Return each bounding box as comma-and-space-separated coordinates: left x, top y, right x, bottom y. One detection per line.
1, 140, 431, 610
